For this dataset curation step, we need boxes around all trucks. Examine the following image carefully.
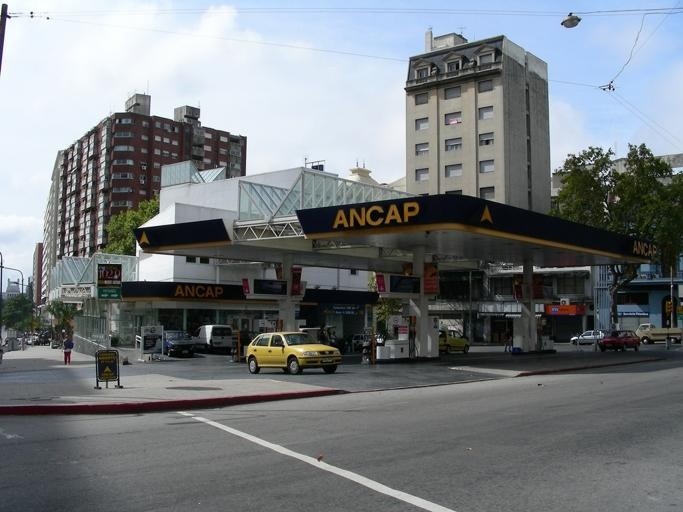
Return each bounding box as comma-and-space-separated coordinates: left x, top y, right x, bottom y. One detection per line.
635, 323, 683, 344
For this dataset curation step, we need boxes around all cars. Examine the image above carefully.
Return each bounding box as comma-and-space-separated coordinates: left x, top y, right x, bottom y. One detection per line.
162, 331, 193, 356
570, 330, 605, 345
598, 330, 640, 351
439, 329, 470, 354
246, 331, 343, 374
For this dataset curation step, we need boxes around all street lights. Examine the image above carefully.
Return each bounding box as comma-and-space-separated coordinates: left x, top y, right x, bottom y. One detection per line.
2, 266, 33, 352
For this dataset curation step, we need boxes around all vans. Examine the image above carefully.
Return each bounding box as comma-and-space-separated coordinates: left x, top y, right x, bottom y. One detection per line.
193, 325, 233, 352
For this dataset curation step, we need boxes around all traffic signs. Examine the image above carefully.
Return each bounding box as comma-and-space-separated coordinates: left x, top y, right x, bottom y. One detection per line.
97, 287, 121, 301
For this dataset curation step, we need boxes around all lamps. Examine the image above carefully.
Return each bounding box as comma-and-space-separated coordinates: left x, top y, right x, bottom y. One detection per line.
561, 12, 582, 28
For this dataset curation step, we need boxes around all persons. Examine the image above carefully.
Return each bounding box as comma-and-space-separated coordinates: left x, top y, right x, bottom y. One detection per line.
63, 335, 71, 365
504, 328, 513, 352
329, 331, 336, 346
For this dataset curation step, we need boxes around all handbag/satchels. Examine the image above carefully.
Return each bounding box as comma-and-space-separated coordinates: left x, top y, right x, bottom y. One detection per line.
68, 340, 73, 348
